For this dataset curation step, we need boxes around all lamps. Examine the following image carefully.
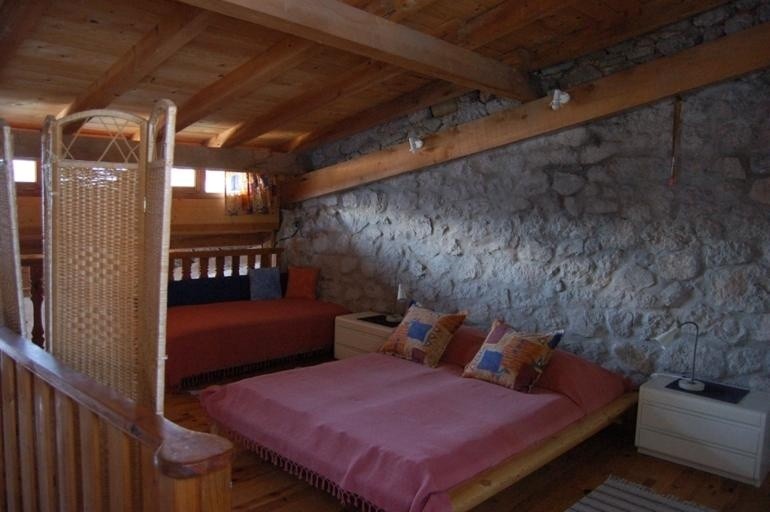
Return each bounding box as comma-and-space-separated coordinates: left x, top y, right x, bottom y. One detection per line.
406, 130, 426, 155
547, 87, 571, 111
646, 319, 709, 392
384, 280, 409, 322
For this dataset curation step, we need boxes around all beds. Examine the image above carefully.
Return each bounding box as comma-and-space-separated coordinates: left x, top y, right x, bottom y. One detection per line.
194, 321, 644, 511
157, 297, 356, 396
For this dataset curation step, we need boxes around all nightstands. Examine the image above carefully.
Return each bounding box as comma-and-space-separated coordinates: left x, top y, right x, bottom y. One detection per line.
333, 309, 404, 363
634, 370, 769, 487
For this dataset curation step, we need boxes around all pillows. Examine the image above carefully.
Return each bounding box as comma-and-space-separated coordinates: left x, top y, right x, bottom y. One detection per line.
373, 302, 470, 369
248, 262, 282, 302
284, 263, 324, 300
461, 315, 564, 397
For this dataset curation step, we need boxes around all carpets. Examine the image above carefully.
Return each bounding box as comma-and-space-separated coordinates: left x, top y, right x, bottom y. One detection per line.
558, 472, 719, 510
